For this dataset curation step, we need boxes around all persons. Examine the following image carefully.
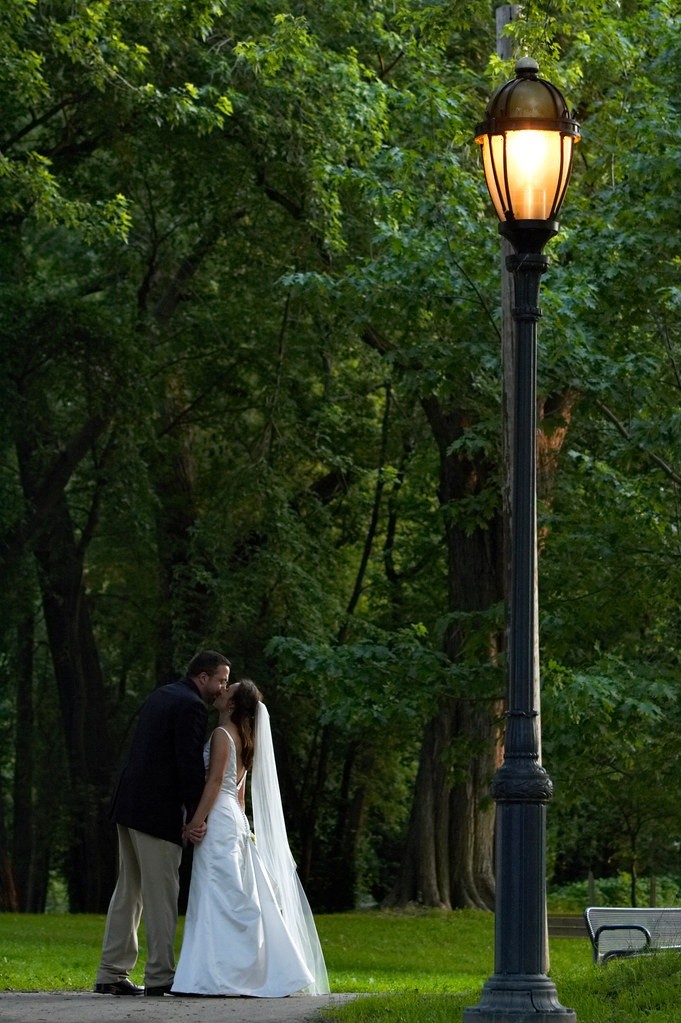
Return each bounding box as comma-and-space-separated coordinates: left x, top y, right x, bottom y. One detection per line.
95, 650, 232, 996
171, 680, 331, 998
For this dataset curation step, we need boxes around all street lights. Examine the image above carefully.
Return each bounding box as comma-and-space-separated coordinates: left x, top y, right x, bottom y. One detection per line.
461, 52, 579, 1023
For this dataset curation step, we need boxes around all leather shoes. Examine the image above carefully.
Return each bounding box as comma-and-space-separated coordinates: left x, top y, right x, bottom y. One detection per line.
94, 978, 144, 995
144, 983, 189, 996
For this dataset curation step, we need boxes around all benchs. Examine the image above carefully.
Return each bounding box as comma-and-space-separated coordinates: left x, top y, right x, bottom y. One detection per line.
585, 906, 681, 966
